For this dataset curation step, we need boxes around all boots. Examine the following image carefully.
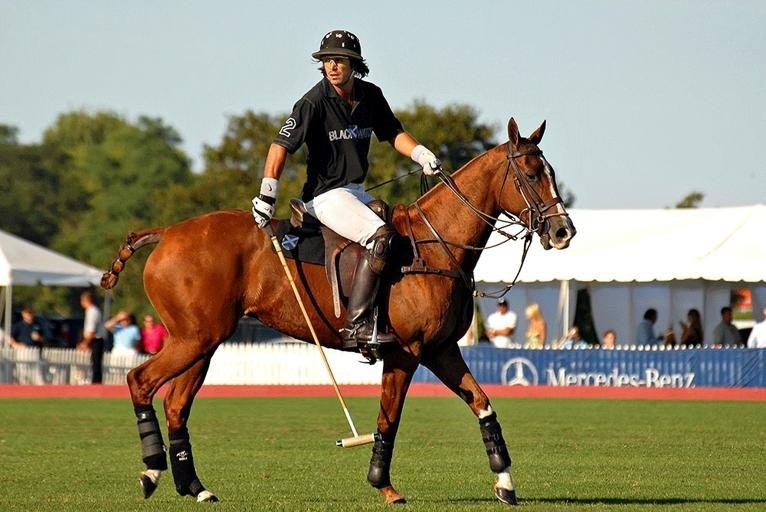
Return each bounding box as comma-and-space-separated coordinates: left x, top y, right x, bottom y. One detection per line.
340, 247, 398, 348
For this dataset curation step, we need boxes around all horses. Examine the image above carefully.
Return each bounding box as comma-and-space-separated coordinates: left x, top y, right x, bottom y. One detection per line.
98, 115, 577, 507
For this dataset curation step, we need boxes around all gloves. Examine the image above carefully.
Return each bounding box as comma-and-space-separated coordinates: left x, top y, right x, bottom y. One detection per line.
410, 143, 444, 176
252, 177, 279, 231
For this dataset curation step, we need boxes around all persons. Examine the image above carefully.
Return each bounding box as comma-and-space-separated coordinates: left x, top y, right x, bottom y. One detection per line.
138, 314, 170, 355
104, 309, 140, 374
251, 31, 440, 349
80, 292, 107, 385
8, 307, 44, 386
484, 287, 766, 347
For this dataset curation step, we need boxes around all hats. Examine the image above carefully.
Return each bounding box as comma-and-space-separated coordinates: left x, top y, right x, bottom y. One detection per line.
312, 29, 363, 60
